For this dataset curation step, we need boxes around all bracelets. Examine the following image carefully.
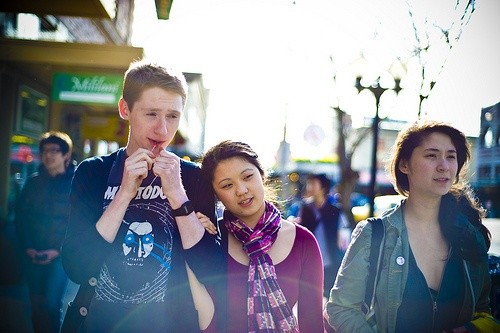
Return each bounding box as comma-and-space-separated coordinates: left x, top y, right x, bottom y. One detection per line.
172, 200, 194, 217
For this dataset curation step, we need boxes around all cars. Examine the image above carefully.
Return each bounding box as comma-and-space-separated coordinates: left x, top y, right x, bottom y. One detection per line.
351, 194, 408, 224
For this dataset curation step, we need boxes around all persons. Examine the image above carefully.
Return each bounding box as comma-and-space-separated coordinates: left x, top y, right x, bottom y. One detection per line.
15, 131, 77, 333
326, 120, 500, 333
185, 140, 324, 333
59, 64, 226, 333
265, 169, 371, 302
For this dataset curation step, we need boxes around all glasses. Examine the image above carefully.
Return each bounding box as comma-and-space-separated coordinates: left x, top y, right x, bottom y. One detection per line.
41, 148, 61, 155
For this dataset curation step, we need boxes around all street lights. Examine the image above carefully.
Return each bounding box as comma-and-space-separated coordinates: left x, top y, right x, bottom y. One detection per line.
352, 53, 408, 218
